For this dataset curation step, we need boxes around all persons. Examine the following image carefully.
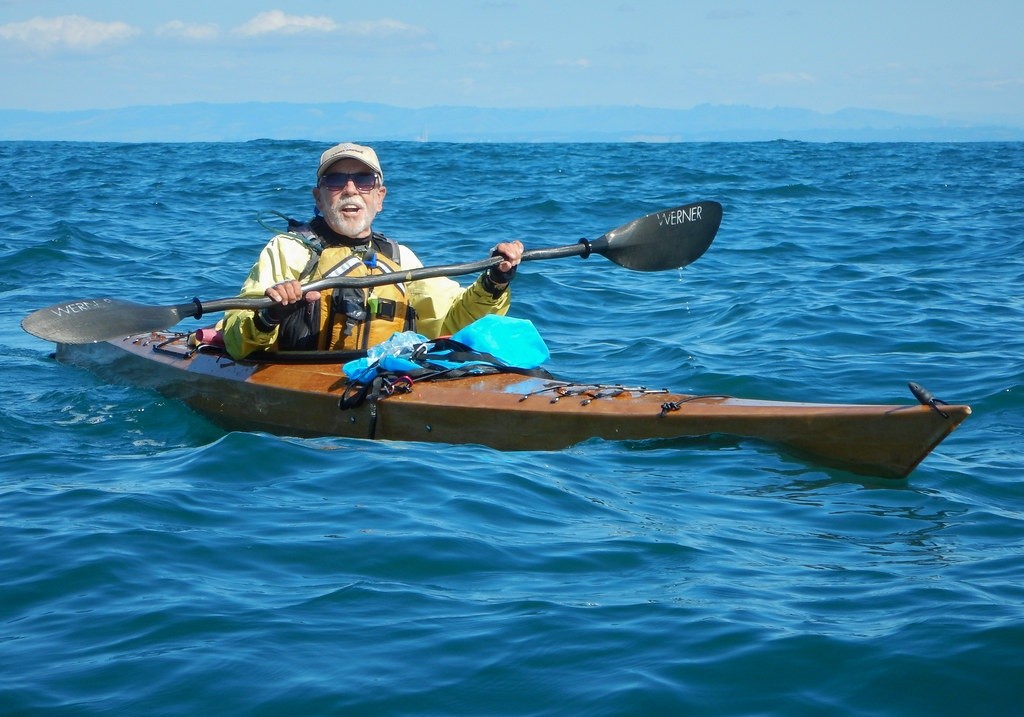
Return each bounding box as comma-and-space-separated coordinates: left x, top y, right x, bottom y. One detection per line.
220, 140, 526, 360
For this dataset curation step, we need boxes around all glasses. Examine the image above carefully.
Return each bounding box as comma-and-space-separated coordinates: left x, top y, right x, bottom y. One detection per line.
317, 172, 378, 191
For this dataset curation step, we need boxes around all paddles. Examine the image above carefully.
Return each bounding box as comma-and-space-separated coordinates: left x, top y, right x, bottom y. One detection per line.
19, 202, 724, 345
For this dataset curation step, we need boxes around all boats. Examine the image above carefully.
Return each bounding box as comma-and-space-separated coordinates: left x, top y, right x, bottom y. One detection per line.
54, 326, 974, 488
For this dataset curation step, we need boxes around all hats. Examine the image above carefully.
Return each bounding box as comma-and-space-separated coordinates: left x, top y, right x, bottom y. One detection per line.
317, 143, 383, 181
